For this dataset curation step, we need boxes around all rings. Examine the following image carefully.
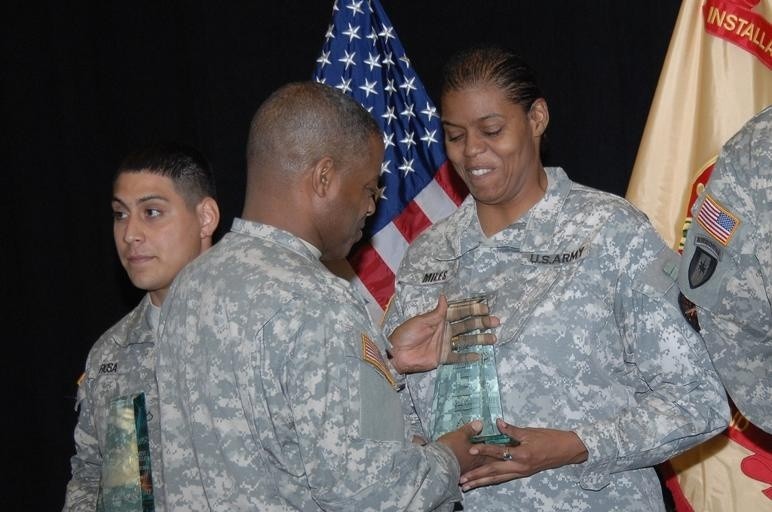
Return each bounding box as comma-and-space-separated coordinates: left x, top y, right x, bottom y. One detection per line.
451, 336, 458, 350
504, 448, 512, 461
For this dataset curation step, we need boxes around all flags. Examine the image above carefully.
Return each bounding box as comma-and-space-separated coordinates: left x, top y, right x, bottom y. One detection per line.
696, 196, 738, 246
626, 1, 770, 512
314, 0, 468, 322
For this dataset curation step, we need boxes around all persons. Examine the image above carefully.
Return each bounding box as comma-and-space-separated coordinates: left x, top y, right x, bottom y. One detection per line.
63, 140, 219, 512
681, 106, 772, 431
157, 82, 498, 512
382, 41, 730, 511
